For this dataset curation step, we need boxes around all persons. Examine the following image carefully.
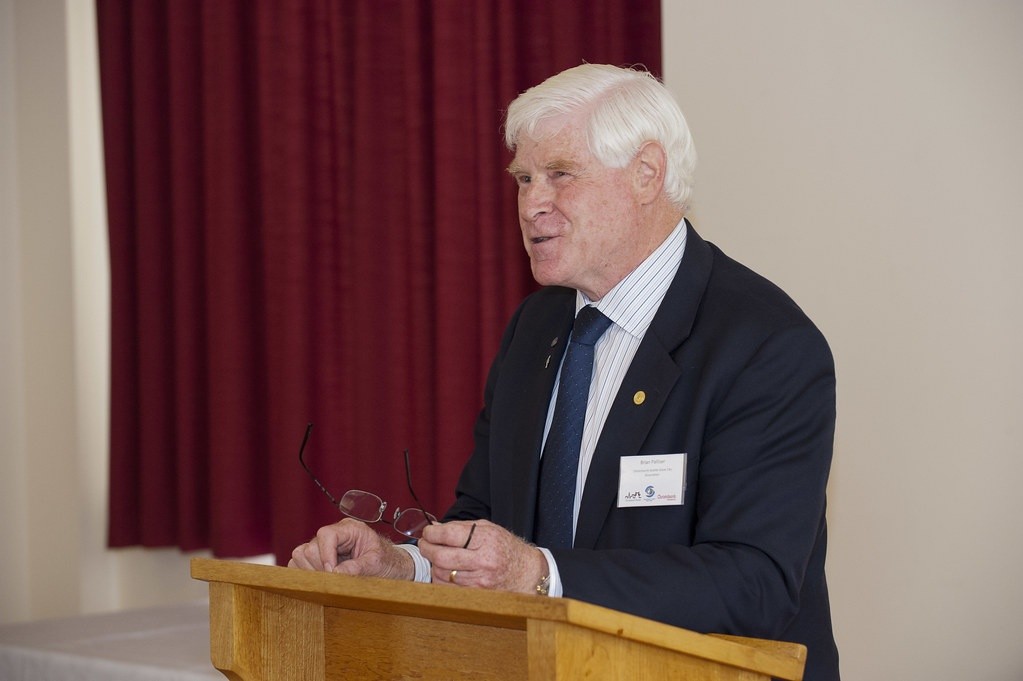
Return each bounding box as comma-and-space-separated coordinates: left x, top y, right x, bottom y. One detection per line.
287, 63, 840, 681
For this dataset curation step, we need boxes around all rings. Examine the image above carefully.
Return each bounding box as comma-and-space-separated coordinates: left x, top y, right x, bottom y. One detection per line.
450, 570, 458, 582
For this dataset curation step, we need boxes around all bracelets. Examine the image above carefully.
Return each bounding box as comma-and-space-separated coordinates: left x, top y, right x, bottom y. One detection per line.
537, 575, 550, 596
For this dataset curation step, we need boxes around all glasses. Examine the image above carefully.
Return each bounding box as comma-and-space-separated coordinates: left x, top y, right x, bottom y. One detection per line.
299, 422, 437, 540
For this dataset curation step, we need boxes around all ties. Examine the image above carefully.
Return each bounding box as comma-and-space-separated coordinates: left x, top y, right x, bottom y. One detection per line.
533, 304, 612, 549
